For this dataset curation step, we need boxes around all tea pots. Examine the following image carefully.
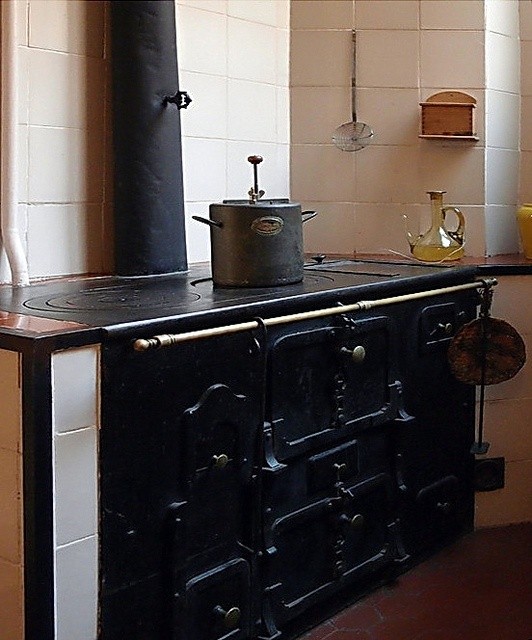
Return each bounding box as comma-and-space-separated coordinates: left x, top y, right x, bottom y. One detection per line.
400, 190, 467, 263
